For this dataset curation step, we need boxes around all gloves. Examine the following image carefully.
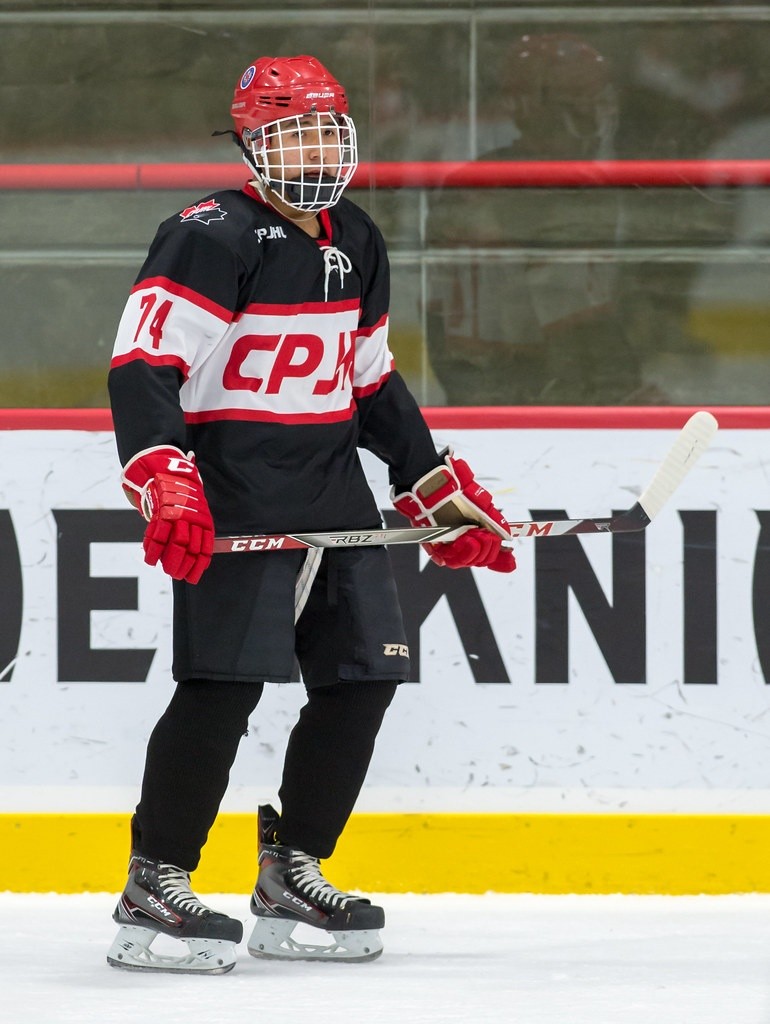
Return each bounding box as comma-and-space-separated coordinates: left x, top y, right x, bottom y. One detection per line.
122, 444, 215, 585
389, 444, 517, 574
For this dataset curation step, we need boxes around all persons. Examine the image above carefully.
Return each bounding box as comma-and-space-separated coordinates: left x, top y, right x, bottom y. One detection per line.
103, 57, 517, 973
422, 34, 668, 405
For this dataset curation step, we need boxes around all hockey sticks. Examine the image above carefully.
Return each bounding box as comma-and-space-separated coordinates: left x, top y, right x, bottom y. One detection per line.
212, 408, 721, 556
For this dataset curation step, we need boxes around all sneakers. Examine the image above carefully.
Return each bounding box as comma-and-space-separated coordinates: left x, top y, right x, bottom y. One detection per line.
247, 804, 385, 964
106, 813, 243, 976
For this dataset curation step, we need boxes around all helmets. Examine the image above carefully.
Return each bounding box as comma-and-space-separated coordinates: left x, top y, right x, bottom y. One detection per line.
210, 55, 359, 212
500, 28, 620, 143
